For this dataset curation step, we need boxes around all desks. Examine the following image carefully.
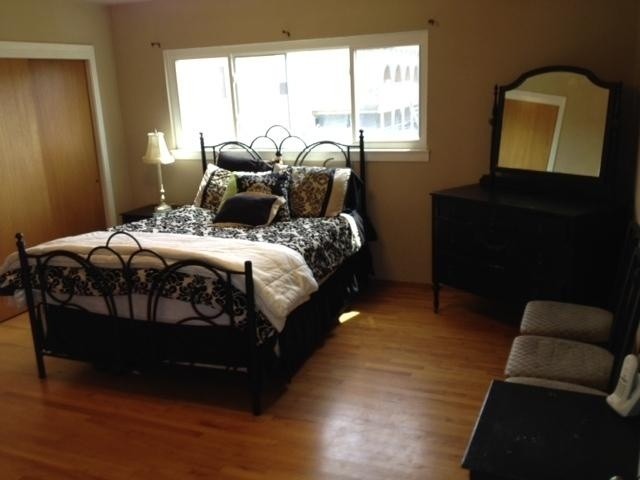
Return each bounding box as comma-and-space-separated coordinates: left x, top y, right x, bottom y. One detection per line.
463, 376, 638, 480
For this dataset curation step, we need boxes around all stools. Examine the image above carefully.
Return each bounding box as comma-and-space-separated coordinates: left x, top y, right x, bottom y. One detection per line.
505, 375, 613, 402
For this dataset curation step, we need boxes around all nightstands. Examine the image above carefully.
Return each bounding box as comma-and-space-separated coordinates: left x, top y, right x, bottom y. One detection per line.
121, 203, 180, 222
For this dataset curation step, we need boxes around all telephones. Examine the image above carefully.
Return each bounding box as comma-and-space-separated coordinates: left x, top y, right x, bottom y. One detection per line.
606, 354, 640, 418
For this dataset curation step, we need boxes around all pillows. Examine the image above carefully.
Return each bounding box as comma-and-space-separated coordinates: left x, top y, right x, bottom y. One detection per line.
191, 165, 231, 211
211, 194, 287, 229
218, 152, 274, 172
273, 159, 353, 218
216, 174, 238, 216
234, 171, 292, 223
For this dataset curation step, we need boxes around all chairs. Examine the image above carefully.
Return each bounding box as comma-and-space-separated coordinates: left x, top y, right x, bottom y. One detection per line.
520, 226, 640, 339
504, 287, 638, 389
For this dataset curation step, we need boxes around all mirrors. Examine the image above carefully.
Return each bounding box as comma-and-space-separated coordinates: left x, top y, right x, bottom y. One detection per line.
489, 65, 623, 189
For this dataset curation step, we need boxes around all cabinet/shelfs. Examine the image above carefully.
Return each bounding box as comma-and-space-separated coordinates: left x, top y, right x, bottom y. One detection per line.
429, 180, 624, 319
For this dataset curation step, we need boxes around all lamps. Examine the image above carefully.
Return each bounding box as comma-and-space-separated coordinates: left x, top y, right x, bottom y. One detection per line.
140, 128, 176, 213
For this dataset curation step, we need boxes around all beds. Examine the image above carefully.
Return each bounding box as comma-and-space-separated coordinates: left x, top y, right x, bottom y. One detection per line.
12, 122, 368, 417
